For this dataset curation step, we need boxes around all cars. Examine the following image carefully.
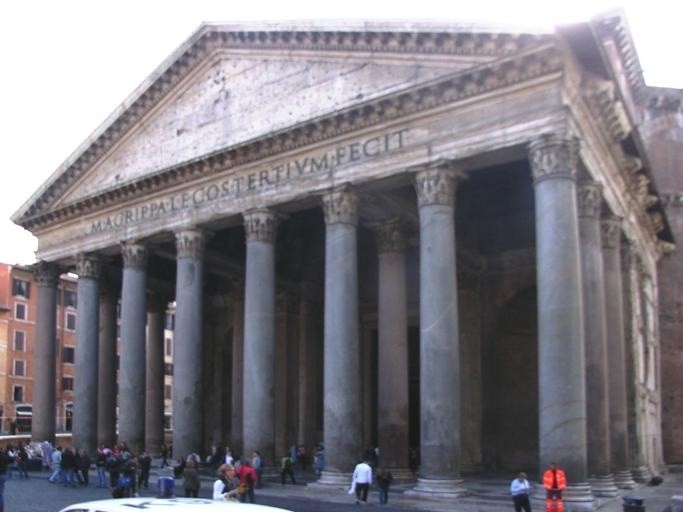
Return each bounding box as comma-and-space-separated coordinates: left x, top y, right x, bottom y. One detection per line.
54, 474, 296, 512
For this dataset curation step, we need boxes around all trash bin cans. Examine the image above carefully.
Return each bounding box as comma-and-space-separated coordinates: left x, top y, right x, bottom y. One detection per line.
622, 495, 645, 512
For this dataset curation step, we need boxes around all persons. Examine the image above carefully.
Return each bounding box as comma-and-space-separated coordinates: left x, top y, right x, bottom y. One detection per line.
0, 439, 323, 512
510, 470, 532, 512
352, 457, 373, 505
542, 463, 567, 512
375, 466, 393, 504
